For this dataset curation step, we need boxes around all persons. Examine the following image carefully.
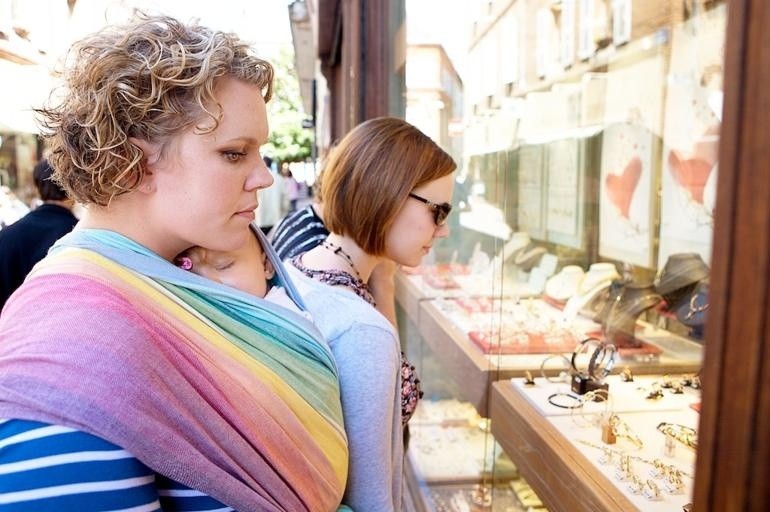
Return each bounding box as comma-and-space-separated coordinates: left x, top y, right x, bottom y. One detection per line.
263, 117, 455, 511
0, 10, 348, 512
176, 221, 315, 323
271, 140, 338, 261
253, 148, 303, 236
0, 159, 79, 317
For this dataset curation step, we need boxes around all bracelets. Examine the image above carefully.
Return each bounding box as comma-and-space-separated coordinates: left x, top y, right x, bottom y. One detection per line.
541, 333, 644, 448
656, 422, 698, 449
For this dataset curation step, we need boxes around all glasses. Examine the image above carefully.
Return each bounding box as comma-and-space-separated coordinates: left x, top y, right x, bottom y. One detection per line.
408, 193, 451, 226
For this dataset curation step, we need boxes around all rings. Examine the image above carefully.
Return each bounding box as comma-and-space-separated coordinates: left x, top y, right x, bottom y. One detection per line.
620, 368, 700, 399
618, 456, 687, 498
525, 370, 534, 383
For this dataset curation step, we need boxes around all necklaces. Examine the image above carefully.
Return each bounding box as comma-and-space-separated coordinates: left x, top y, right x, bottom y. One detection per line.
524, 493, 541, 501
574, 273, 621, 297
514, 246, 545, 265
604, 290, 662, 333
656, 260, 700, 289
319, 239, 365, 284
683, 291, 710, 322
